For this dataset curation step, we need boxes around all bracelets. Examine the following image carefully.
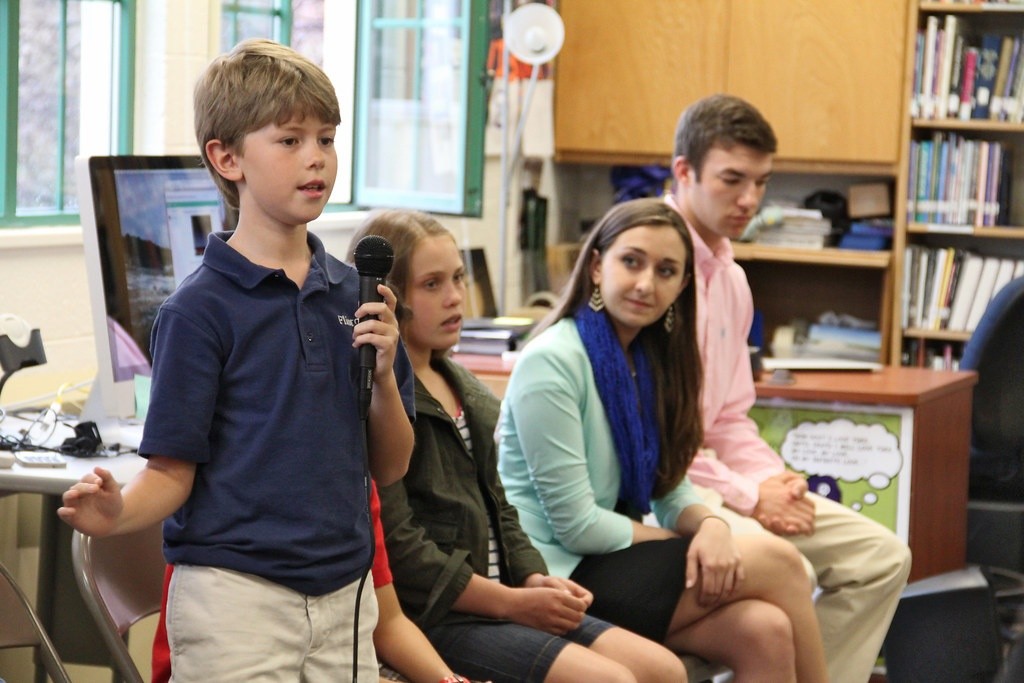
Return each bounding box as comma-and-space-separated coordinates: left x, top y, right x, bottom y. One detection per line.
698, 515, 733, 528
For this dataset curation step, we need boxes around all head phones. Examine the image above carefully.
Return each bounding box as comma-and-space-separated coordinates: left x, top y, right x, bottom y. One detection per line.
58, 421, 101, 458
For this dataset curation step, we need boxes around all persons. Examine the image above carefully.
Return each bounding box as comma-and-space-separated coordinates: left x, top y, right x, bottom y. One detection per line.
663, 93, 912, 683
496, 199, 829, 683
345, 208, 688, 682
152, 474, 464, 683
54, 37, 415, 683
952, 273, 1024, 683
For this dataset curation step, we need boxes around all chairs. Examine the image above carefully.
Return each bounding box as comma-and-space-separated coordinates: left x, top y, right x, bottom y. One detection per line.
954, 277, 1024, 645
72, 518, 165, 683
1, 564, 73, 683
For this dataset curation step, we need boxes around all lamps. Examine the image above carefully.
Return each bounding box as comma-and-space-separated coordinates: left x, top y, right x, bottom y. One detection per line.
494, 0, 565, 317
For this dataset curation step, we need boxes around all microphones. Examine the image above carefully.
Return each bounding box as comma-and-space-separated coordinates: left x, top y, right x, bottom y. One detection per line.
353, 236, 394, 421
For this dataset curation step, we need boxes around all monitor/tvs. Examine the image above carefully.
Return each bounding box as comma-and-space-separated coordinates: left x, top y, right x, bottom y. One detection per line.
73, 155, 240, 418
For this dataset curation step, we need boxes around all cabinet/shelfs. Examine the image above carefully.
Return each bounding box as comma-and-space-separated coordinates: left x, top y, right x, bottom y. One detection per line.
548, 0, 1024, 370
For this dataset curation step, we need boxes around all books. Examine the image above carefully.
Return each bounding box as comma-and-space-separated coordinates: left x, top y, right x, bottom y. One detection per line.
912, 12, 1024, 124
905, 244, 1024, 335
905, 340, 964, 376
742, 205, 831, 249
907, 129, 1005, 231
463, 315, 538, 354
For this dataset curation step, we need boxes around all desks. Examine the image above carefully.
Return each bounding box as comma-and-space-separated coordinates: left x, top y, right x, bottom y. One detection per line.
0, 403, 150, 682
470, 368, 979, 683
